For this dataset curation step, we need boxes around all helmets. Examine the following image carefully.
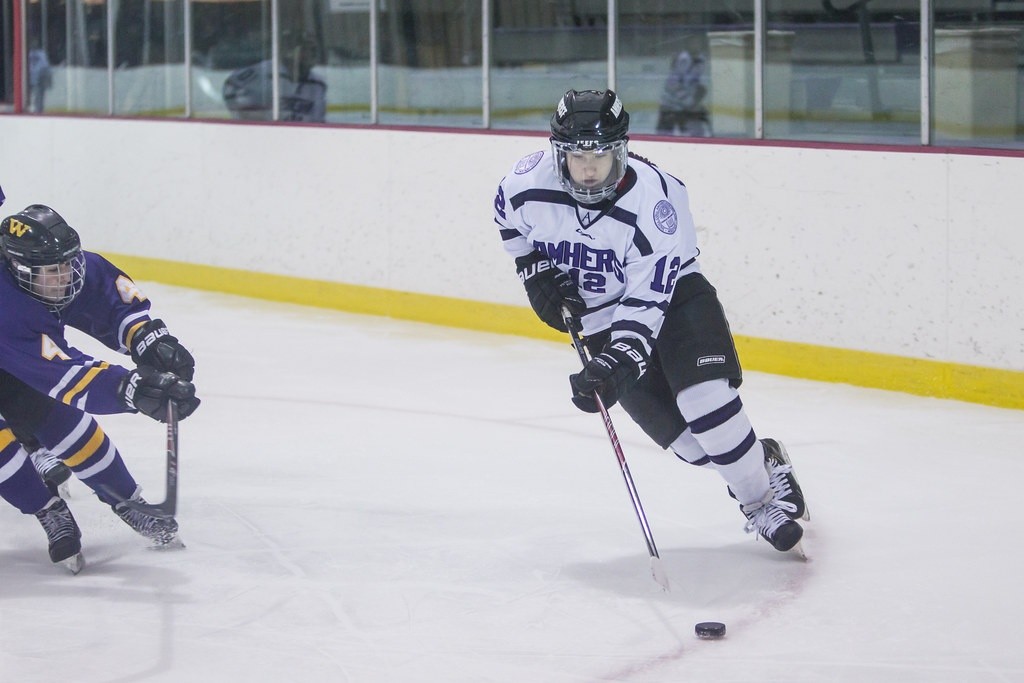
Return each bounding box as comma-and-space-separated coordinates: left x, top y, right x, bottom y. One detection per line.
549, 89, 631, 152
0, 203, 81, 273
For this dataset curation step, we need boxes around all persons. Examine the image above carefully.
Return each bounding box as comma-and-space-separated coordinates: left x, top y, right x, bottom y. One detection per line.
493, 88, 811, 563
0, 204, 201, 575
654, 36, 715, 137
224, 27, 327, 123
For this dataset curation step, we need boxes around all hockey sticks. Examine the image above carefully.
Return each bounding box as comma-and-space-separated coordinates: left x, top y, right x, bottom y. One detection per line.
562, 304, 670, 595
98, 395, 182, 523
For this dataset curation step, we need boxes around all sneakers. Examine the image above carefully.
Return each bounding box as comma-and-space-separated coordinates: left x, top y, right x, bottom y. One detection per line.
28, 445, 75, 499
34, 500, 86, 576
739, 494, 808, 561
758, 438, 810, 522
112, 496, 188, 549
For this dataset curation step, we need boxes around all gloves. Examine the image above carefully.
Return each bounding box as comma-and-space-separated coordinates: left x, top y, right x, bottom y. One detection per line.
569, 337, 648, 414
130, 318, 195, 382
515, 258, 586, 333
117, 365, 202, 422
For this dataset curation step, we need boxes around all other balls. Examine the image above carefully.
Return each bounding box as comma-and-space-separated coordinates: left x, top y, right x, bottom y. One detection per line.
695, 619, 727, 639
